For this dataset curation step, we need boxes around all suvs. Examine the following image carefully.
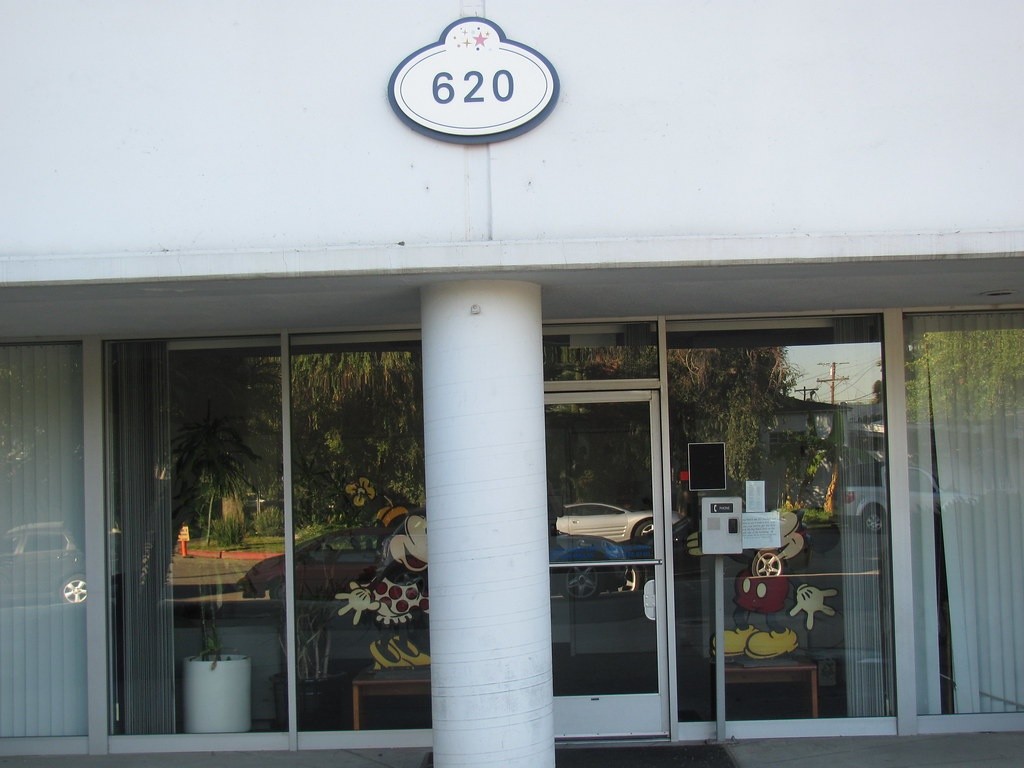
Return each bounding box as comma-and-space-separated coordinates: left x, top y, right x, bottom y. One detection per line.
843, 461, 981, 536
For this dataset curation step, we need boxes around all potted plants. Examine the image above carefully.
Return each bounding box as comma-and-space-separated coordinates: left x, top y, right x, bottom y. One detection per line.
269, 574, 349, 734
166, 349, 284, 733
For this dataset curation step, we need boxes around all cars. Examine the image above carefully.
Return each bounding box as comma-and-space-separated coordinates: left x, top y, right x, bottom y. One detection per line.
548, 501, 691, 603
0, 520, 90, 608
237, 527, 397, 600
